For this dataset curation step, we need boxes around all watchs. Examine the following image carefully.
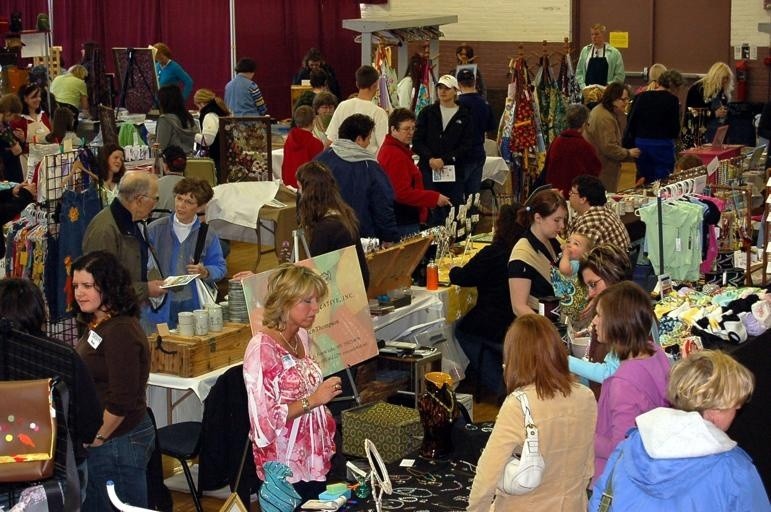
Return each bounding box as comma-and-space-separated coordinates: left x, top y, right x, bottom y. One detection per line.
302, 395, 311, 413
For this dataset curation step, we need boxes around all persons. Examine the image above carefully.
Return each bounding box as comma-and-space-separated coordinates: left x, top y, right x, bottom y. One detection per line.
0, 276, 98, 510
449, 202, 529, 394
81, 169, 169, 304
296, 163, 370, 298
587, 349, 771, 511
597, 279, 681, 486
143, 39, 274, 151
567, 182, 631, 253
469, 313, 596, 511
243, 264, 342, 512
65, 249, 160, 510
0, 64, 91, 213
558, 233, 592, 330
148, 175, 229, 330
507, 188, 596, 337
542, 16, 741, 202
104, 143, 129, 205
568, 244, 659, 384
293, 39, 496, 232
144, 143, 190, 216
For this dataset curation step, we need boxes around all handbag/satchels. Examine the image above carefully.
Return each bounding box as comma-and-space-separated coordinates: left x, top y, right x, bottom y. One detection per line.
496, 389, 544, 497
258, 460, 302, 512
193, 221, 219, 310
194, 135, 209, 158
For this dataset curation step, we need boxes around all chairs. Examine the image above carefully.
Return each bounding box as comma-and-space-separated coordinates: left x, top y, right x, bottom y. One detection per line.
0, 374, 70, 488
143, 358, 259, 511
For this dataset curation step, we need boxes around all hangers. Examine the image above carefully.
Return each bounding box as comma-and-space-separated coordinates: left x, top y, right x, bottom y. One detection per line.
353, 26, 449, 50
3, 204, 65, 243
507, 40, 589, 75
634, 177, 728, 221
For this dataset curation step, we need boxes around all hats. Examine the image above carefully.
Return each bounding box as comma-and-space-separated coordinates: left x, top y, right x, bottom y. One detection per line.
435, 74, 458, 90
158, 145, 187, 168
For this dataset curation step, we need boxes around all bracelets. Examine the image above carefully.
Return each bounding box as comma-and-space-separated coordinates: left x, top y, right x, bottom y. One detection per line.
95, 428, 108, 440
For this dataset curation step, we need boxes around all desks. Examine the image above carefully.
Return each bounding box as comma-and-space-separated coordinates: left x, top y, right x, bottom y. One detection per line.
467, 229, 503, 245
557, 284, 771, 385
14, 63, 341, 265
478, 155, 513, 214
337, 447, 520, 511
418, 235, 507, 326
147, 280, 477, 495
202, 177, 307, 275
678, 142, 750, 192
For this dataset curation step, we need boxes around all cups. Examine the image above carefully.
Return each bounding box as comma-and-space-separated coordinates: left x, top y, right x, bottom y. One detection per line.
208, 304, 224, 333
217, 294, 231, 319
608, 193, 649, 217
178, 311, 194, 336
193, 310, 209, 336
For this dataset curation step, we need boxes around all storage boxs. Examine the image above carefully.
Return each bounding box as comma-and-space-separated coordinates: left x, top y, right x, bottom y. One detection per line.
143, 316, 258, 381
337, 396, 431, 466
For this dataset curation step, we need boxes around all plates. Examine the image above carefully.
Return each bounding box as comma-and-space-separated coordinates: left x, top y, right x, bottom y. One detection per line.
229, 277, 251, 322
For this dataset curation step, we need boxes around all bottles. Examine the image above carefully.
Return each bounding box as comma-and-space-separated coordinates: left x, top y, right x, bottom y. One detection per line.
427, 257, 439, 290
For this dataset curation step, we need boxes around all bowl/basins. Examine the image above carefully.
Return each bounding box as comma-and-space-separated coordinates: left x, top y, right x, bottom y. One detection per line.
572, 337, 592, 358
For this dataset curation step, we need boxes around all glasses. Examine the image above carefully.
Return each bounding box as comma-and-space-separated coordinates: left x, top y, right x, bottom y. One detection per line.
569, 189, 579, 195
135, 194, 159, 202
584, 278, 603, 292
618, 96, 630, 102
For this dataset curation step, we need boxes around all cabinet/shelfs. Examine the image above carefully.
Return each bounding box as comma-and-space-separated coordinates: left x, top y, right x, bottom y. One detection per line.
763, 165, 771, 290
378, 341, 445, 410
